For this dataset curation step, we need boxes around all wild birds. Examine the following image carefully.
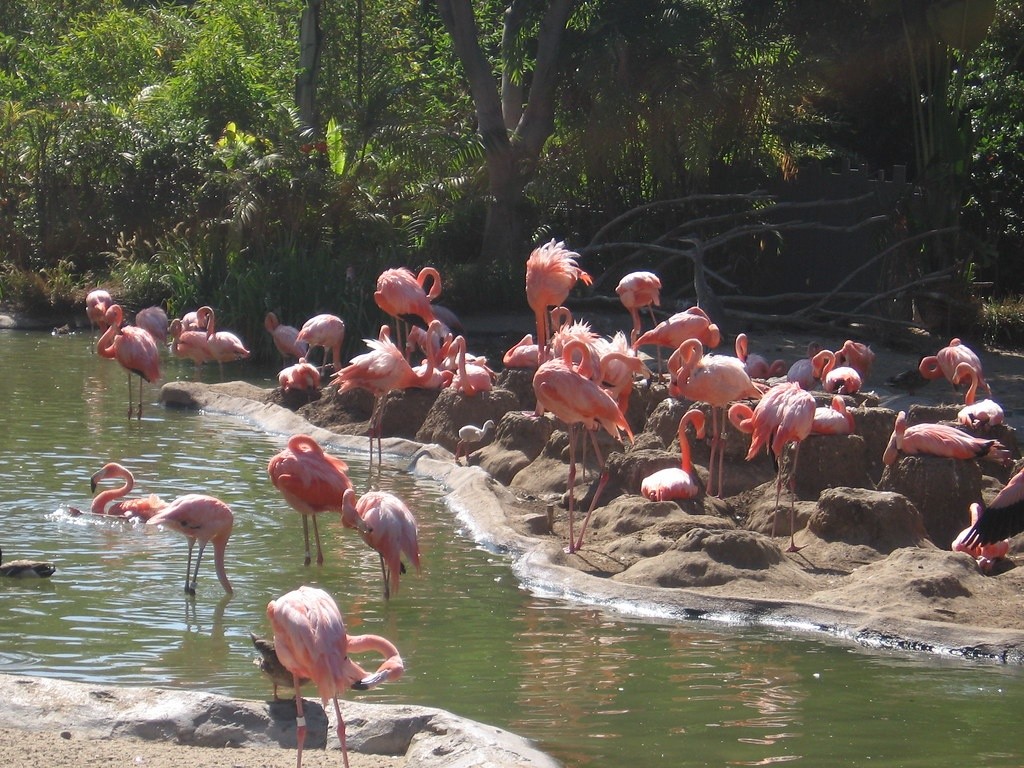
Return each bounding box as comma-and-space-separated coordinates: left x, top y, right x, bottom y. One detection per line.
84, 237, 1024, 576
87, 433, 421, 768
